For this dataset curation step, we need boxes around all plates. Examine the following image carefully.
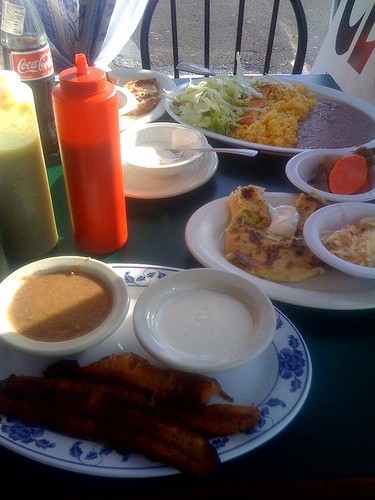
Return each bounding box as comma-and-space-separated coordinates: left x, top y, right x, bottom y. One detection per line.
184, 192, 375, 310
164, 76, 375, 156
1, 261, 314, 476
106, 68, 177, 130
122, 144, 217, 199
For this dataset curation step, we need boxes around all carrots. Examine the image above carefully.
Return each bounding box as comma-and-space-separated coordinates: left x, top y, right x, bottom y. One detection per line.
328, 155, 370, 195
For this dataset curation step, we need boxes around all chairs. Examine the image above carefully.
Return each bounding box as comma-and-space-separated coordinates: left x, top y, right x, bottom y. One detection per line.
139, 0, 308, 79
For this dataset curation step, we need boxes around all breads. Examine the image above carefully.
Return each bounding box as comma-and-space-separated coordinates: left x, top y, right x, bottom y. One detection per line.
122, 77, 159, 116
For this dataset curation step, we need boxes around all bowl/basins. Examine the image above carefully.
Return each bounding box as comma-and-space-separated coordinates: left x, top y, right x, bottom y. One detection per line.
120, 122, 208, 179
0, 255, 130, 356
132, 269, 276, 375
284, 149, 375, 202
302, 202, 375, 280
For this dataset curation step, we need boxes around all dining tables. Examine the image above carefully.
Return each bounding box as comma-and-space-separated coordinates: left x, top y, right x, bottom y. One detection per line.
0, 74, 375, 500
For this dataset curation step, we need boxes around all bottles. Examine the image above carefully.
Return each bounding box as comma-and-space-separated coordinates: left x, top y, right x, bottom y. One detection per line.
0, 68, 59, 259
52, 54, 128, 254
0, 0, 59, 156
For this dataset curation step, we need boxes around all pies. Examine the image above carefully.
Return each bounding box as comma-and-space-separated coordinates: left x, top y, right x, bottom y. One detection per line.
223, 184, 332, 283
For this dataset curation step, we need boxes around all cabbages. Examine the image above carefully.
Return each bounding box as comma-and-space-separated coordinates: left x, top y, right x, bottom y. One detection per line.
160, 50, 277, 136
